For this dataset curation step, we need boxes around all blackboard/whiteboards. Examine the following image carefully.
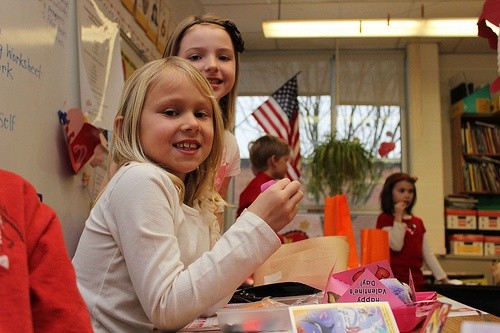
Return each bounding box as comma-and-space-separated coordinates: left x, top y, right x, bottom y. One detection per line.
0, 0, 146, 261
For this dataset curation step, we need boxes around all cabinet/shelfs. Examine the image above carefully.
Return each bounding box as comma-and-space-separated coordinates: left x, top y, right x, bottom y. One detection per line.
452, 113, 500, 197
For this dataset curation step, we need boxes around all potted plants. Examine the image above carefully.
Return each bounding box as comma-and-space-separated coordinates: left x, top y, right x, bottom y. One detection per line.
305, 136, 384, 205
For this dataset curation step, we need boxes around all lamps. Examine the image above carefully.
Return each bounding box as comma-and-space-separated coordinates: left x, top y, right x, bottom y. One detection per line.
262, 0, 478, 38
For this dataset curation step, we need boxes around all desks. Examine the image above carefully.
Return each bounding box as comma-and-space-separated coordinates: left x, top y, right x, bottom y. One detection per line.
406, 293, 500, 332
